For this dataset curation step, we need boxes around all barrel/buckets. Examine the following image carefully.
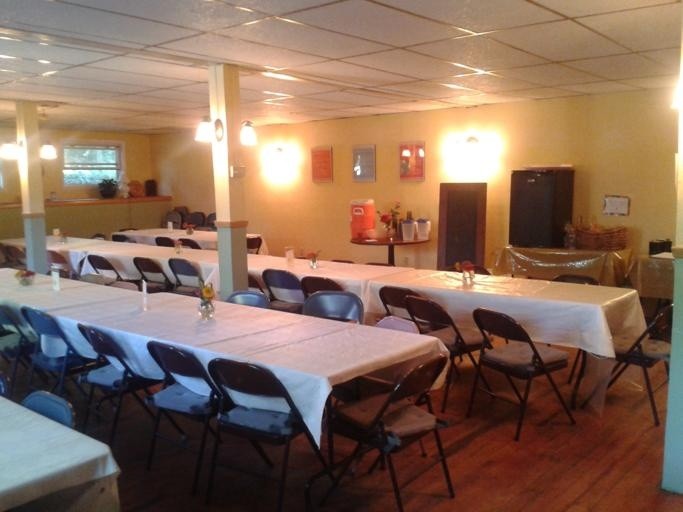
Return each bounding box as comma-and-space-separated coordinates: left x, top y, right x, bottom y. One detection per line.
417, 218, 431, 241
401, 220, 418, 242
349, 199, 376, 239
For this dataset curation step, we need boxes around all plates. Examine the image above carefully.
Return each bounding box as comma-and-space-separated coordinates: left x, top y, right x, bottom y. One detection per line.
648, 238, 672, 256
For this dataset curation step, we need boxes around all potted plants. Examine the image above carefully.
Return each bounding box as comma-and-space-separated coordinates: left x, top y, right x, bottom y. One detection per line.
98, 179, 118, 199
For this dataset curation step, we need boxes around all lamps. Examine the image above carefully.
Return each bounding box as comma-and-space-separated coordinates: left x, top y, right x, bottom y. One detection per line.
196, 116, 224, 143
40, 141, 56, 158
240, 120, 257, 145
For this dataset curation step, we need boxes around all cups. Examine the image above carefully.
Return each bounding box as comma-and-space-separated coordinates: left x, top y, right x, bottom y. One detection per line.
400, 218, 431, 241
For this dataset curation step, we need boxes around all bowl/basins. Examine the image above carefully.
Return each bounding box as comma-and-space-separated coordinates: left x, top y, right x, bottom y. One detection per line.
576, 224, 627, 250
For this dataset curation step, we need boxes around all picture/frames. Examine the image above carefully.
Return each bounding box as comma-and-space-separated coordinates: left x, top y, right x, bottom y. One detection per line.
603, 195, 630, 216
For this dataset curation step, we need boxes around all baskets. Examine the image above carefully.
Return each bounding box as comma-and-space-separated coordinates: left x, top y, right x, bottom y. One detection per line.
565, 214, 628, 249
